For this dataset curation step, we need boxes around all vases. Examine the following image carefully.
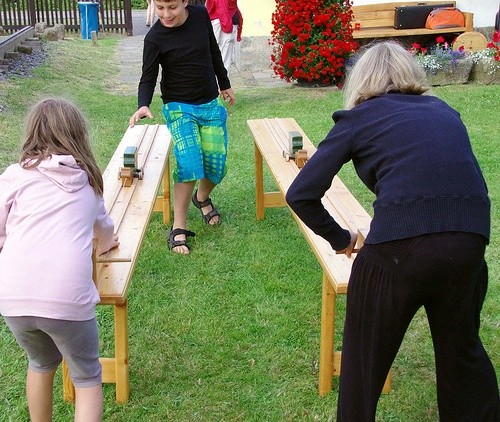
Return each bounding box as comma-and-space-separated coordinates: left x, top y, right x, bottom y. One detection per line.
473, 58, 500, 85
421, 56, 472, 86
299, 78, 331, 87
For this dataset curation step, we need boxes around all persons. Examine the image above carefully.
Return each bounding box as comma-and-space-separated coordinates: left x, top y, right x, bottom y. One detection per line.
0, 98, 120, 422
129, 0, 235, 257
286, 42, 499, 422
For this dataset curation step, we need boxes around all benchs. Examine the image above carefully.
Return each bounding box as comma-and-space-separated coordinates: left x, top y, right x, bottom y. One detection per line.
350, 1, 474, 38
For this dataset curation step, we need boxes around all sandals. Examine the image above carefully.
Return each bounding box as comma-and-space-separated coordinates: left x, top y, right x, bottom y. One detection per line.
192, 188, 223, 226
167, 224, 195, 254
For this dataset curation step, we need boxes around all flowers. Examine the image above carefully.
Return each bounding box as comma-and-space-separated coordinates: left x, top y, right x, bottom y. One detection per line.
404, 35, 472, 74
266, 0, 360, 88
470, 30, 500, 76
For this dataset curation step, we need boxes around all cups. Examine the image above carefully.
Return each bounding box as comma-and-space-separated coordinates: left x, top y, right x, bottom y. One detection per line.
355, 23, 360, 31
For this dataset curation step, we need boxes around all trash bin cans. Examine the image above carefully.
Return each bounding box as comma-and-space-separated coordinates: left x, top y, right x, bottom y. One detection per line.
77, 1, 101, 39
